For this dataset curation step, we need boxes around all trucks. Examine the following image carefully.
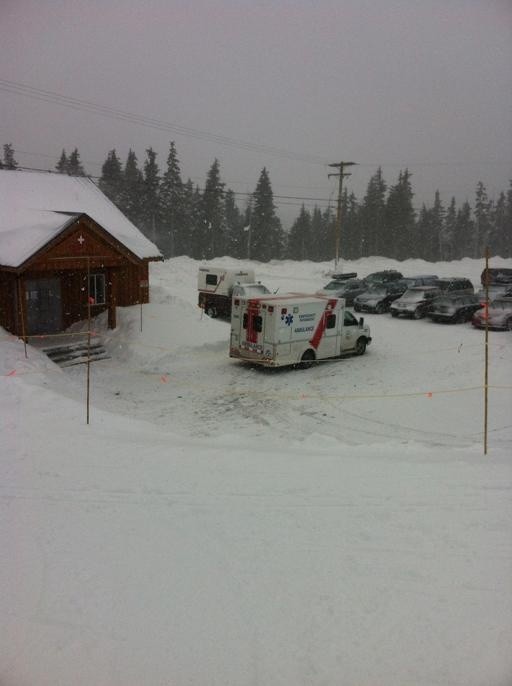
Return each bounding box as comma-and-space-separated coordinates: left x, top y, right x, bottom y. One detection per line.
196, 265, 272, 323
229, 291, 373, 370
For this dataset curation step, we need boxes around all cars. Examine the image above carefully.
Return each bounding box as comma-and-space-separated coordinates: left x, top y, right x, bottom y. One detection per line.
315, 267, 512, 332
